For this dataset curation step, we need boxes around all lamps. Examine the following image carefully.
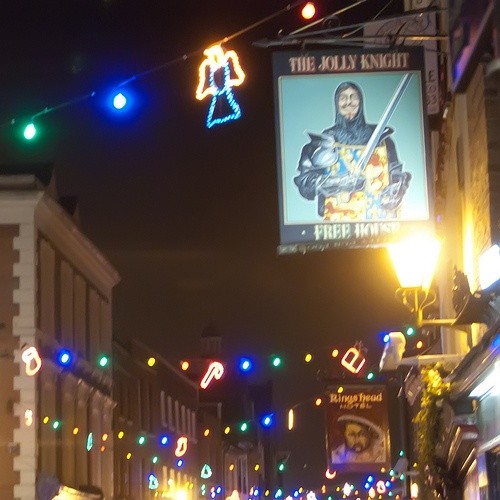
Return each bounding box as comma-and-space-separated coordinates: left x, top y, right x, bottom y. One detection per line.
388, 231, 473, 332
